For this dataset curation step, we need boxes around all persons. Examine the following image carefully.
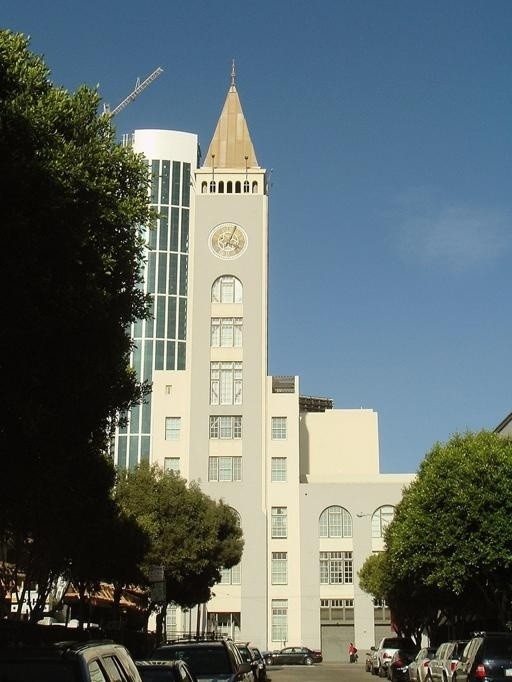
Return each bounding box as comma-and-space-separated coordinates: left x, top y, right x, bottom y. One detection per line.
349, 643, 357, 663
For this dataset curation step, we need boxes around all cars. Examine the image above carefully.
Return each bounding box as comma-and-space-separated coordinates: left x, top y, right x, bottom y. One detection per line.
0, 637, 268, 681
264, 646, 323, 665
364, 635, 512, 682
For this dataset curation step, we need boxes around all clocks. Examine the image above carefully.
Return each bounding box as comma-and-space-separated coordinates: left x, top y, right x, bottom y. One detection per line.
207, 220, 248, 260
